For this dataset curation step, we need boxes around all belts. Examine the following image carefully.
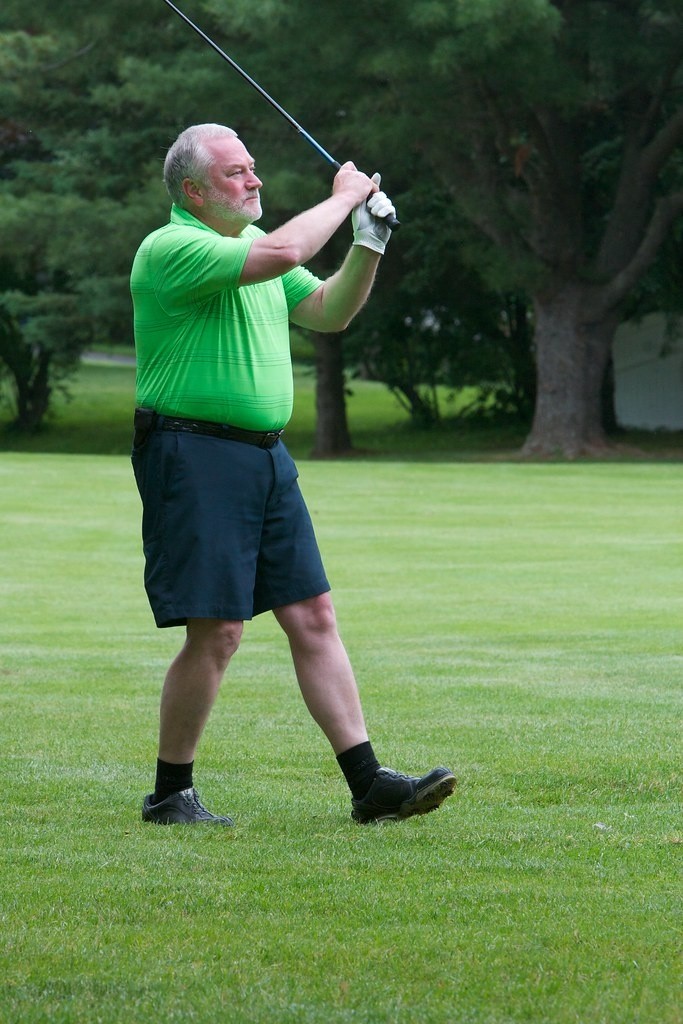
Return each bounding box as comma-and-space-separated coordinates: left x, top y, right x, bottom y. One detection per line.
155, 415, 284, 450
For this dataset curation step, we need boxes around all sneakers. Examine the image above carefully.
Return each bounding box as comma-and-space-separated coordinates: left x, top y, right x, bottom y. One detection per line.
142, 786, 234, 827
351, 766, 457, 825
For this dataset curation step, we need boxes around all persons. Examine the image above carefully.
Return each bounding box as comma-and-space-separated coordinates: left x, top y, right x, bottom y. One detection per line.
130, 124, 456, 828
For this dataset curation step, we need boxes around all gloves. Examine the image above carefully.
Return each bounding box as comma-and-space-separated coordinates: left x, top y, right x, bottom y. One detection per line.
352, 173, 396, 255
